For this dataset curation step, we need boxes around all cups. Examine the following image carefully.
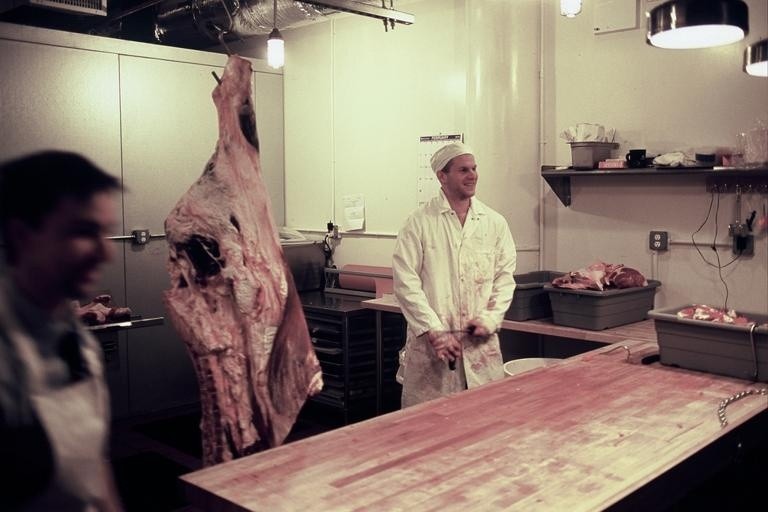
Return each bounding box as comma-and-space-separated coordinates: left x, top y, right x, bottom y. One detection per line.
625, 148, 646, 169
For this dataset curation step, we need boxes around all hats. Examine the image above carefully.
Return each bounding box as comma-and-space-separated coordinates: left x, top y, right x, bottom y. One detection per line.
429, 141, 473, 175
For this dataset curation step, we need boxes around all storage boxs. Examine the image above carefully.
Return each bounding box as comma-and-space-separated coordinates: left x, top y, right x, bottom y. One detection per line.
648, 301, 767, 385
568, 140, 619, 168
543, 277, 662, 331
504, 270, 571, 323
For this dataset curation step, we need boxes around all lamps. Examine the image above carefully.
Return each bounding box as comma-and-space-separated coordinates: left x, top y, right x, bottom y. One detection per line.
266, 0, 285, 70
742, 37, 768, 79
644, 1, 750, 50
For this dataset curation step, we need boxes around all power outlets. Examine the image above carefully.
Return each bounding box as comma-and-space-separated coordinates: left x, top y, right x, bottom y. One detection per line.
649, 231, 668, 252
328, 224, 339, 239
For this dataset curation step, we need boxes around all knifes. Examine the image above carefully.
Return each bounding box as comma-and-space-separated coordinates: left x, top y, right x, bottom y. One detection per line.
447, 311, 458, 374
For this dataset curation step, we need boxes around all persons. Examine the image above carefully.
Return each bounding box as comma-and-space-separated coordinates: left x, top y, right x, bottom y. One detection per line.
392, 141, 517, 410
0, 149, 170, 512
